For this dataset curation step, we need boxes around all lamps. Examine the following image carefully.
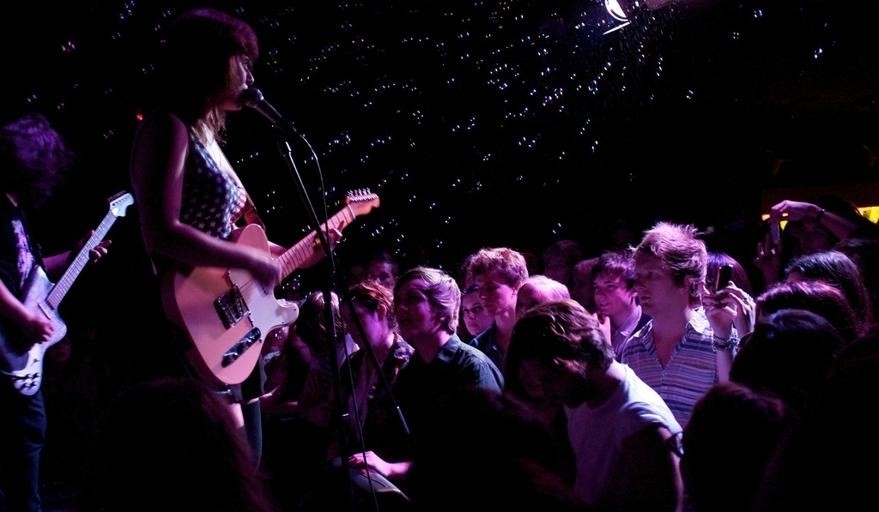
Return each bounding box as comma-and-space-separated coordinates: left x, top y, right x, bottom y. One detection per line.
601, 0, 676, 35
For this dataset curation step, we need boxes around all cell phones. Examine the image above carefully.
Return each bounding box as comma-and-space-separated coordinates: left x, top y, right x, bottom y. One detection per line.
715, 264, 733, 308
769, 214, 780, 245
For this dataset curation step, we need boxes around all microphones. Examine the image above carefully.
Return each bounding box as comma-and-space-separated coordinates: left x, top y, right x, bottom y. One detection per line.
239, 87, 282, 128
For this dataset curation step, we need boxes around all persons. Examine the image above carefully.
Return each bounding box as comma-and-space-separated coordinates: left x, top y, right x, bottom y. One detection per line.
131, 7, 346, 510
247, 196, 879, 511
0, 107, 116, 511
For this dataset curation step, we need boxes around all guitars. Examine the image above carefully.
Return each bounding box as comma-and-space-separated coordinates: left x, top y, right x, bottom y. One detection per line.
161, 188, 381, 386
0, 190, 135, 397
